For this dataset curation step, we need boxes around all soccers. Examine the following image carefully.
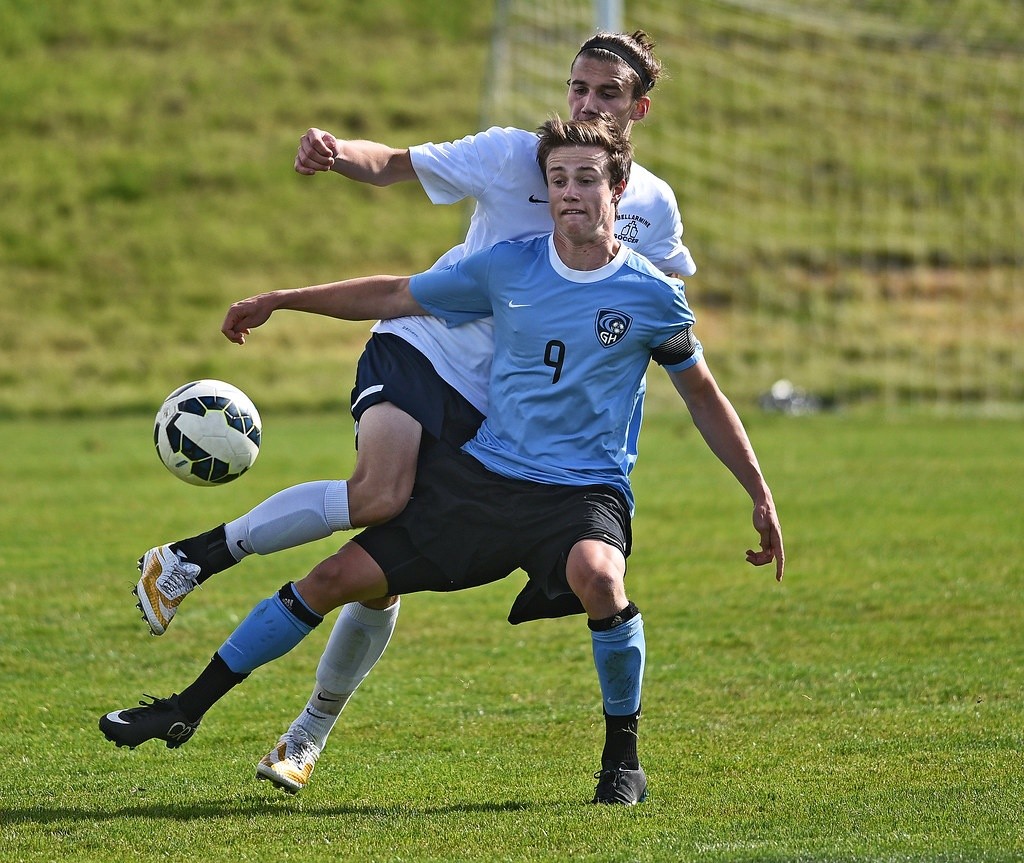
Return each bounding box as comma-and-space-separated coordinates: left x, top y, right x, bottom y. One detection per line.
150, 377, 263, 487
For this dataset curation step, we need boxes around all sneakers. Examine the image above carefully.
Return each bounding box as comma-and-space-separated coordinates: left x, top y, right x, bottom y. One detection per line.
592, 764, 650, 806
131, 542, 200, 636
256, 727, 320, 792
96, 692, 202, 751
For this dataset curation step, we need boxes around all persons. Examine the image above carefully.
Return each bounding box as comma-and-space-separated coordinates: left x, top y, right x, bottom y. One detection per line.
133, 29, 697, 796
98, 111, 785, 751
